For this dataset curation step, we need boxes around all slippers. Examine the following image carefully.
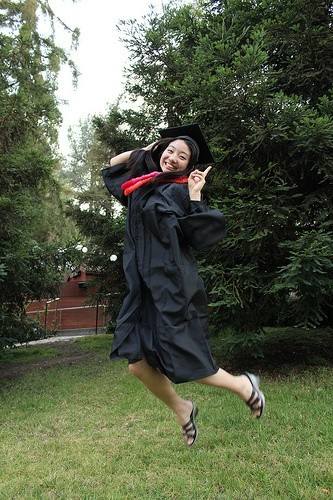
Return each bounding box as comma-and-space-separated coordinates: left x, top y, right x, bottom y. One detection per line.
240, 371, 265, 419
182, 399, 199, 445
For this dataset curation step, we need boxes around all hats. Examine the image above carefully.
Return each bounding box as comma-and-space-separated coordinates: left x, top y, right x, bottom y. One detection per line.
158, 123, 215, 166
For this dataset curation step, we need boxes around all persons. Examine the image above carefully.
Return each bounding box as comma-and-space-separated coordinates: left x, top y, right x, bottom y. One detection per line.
101, 124, 265, 446
